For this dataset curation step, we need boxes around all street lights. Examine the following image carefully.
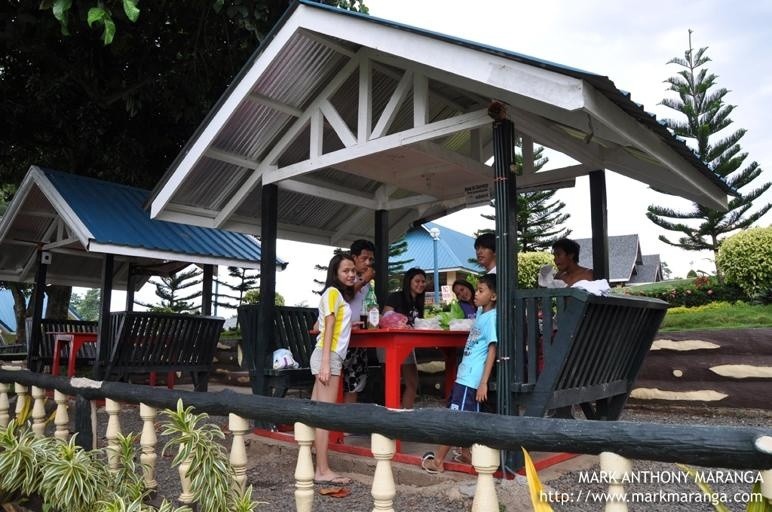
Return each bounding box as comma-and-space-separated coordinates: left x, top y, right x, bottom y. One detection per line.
429, 228, 440, 312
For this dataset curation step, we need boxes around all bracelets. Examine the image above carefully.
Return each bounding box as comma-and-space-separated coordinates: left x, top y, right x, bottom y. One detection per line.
359, 277, 367, 287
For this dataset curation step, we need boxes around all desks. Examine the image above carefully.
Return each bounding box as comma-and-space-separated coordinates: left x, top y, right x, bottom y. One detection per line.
46, 331, 176, 389
328, 328, 470, 455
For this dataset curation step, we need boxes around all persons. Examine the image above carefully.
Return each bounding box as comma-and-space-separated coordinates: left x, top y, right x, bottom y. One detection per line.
304, 252, 358, 489
472, 231, 497, 276
422, 272, 499, 473
308, 238, 377, 437
450, 277, 477, 376
374, 267, 429, 411
533, 237, 595, 378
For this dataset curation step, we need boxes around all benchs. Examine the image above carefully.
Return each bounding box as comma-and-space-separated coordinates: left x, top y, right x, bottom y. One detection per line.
25, 318, 145, 384
238, 304, 384, 432
101, 310, 225, 393
486, 288, 672, 474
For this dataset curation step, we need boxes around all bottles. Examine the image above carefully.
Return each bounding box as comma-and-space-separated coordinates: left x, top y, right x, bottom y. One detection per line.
359, 286, 381, 330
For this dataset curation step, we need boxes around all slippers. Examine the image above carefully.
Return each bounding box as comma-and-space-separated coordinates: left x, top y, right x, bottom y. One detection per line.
420, 450, 440, 474
314, 474, 355, 486
452, 445, 472, 466
